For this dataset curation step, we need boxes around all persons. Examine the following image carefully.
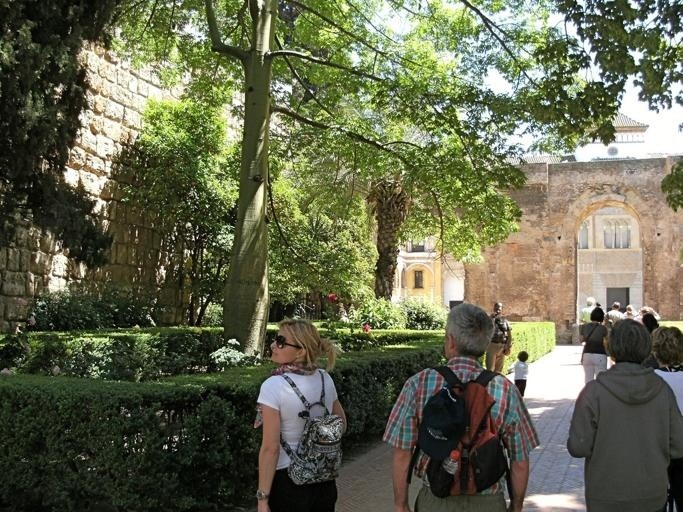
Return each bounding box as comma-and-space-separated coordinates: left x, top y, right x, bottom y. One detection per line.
608, 299, 659, 334
566, 319, 682, 511
485, 302, 511, 373
579, 297, 595, 329
508, 351, 528, 398
579, 307, 608, 384
252, 319, 346, 511
649, 325, 682, 512
596, 302, 602, 308
383, 301, 538, 512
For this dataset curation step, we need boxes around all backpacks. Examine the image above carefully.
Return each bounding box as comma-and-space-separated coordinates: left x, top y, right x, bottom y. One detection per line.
418, 367, 508, 498
280, 369, 347, 485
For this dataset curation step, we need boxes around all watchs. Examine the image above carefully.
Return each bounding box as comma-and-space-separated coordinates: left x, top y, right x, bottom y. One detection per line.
256, 489, 270, 499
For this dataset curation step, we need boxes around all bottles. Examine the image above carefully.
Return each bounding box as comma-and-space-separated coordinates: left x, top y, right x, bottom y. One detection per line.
431, 450, 461, 498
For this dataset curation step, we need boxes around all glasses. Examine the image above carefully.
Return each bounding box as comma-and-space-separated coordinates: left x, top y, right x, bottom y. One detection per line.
274, 333, 302, 349
